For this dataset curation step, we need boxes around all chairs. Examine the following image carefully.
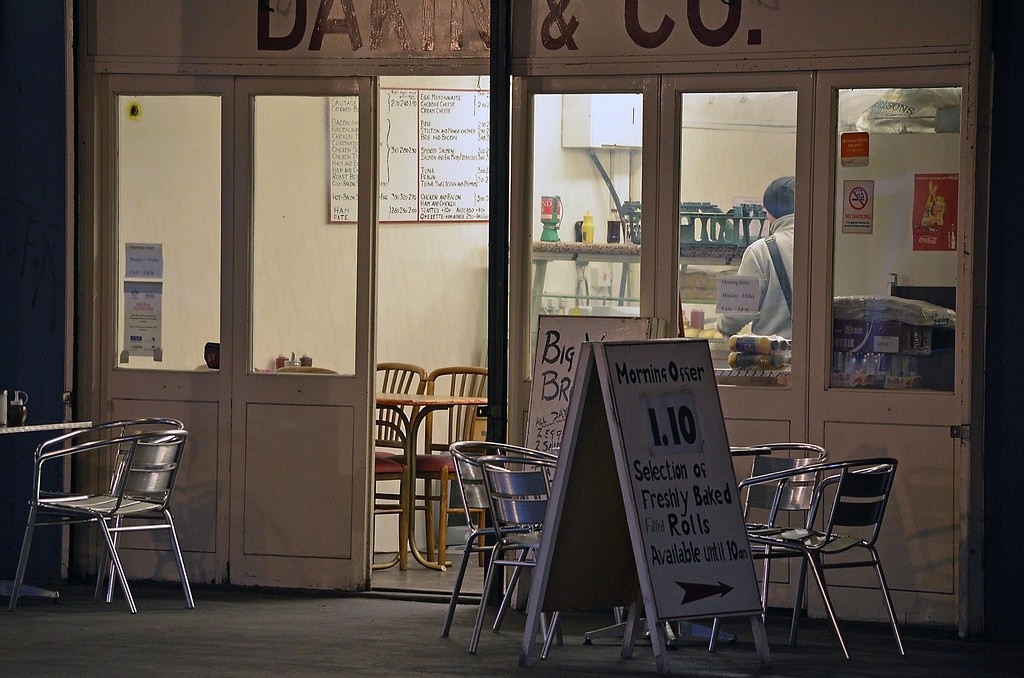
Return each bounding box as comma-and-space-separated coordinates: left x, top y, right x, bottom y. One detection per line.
730, 451, 906, 660
371, 355, 427, 572
7, 415, 197, 617
387, 365, 492, 568
685, 438, 829, 657
439, 434, 571, 657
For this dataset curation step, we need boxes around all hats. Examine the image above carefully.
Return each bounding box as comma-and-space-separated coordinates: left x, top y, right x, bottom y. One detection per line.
763, 176, 794, 219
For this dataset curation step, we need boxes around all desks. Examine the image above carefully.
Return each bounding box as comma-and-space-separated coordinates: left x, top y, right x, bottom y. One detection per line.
0, 420, 94, 602
363, 392, 488, 576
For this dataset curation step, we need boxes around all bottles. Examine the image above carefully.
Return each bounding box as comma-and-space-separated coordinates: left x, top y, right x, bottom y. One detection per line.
726, 335, 790, 368
607, 209, 622, 243
274, 350, 313, 368
575, 211, 595, 244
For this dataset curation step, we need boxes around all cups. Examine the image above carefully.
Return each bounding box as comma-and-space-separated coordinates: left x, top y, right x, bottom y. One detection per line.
0, 389, 8, 426
692, 308, 704, 330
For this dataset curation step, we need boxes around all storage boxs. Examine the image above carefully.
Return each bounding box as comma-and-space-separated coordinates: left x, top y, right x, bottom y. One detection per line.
828, 318, 934, 358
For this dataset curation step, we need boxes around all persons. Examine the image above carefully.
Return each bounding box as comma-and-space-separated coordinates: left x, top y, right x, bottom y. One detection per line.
716, 176, 794, 339
921, 181, 945, 226
